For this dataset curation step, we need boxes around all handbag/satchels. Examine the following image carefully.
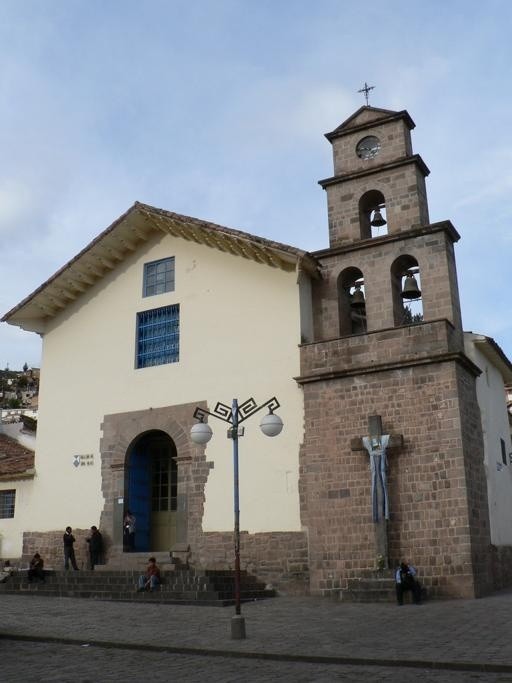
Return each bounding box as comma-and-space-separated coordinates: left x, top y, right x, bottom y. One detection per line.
399, 570, 416, 592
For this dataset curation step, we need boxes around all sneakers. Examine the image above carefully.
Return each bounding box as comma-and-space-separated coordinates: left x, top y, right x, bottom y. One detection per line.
399, 599, 403, 605
137, 587, 155, 592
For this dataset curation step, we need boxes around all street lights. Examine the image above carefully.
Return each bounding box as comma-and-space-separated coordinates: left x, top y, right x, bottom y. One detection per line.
190, 396, 284, 639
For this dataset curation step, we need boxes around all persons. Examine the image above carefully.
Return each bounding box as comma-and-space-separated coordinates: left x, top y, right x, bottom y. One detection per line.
123, 510, 137, 552
395, 563, 421, 605
85, 526, 105, 570
0, 560, 16, 584
137, 557, 160, 593
62, 527, 80, 570
27, 553, 46, 584
